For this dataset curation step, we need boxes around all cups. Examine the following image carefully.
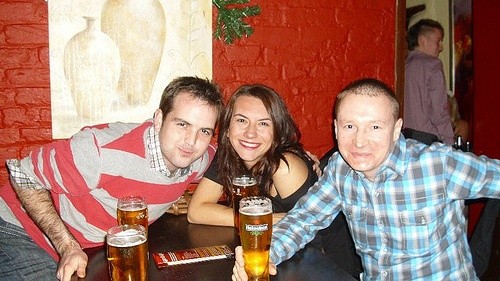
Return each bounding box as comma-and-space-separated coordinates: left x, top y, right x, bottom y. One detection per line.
117, 195, 148, 238
232, 175, 259, 245
106, 224, 149, 281
238, 196, 273, 281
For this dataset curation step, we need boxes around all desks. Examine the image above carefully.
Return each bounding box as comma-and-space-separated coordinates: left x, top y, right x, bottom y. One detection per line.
71, 203, 357, 281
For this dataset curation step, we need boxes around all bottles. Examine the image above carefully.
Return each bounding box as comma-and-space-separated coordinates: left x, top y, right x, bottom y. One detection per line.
456, 135, 463, 151
464, 141, 471, 152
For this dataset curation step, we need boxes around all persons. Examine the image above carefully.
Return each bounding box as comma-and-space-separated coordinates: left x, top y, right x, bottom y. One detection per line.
187, 84, 365, 281
401, 19, 471, 148
0, 76, 323, 281
232, 79, 500, 281
